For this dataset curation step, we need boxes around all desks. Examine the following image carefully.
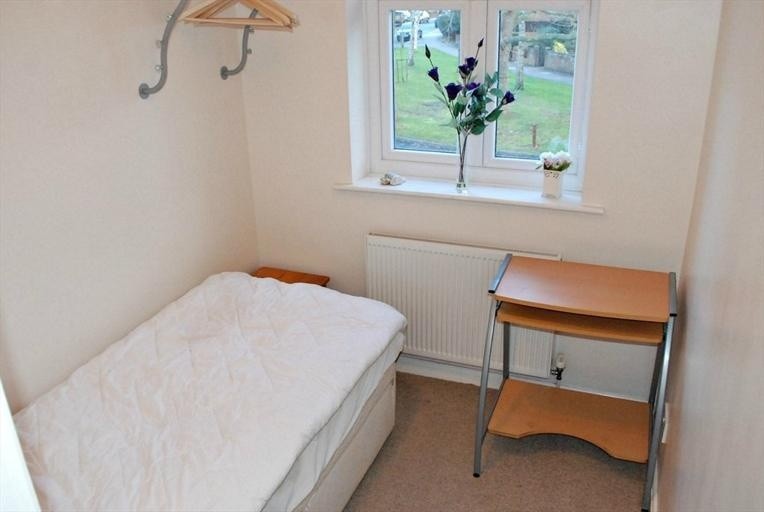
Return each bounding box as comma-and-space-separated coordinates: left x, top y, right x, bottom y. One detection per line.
474, 253, 678, 512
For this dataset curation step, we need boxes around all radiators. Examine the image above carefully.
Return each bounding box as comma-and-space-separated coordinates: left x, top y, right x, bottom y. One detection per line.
365, 233, 562, 379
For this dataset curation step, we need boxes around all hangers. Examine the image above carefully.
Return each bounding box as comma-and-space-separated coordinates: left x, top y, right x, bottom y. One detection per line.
179, 1, 296, 32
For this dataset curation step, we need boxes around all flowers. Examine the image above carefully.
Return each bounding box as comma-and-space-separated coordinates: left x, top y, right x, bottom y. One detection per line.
535, 150, 572, 171
424, 37, 515, 187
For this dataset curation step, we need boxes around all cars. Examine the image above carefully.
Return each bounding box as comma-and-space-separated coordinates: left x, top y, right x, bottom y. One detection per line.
393, 10, 430, 41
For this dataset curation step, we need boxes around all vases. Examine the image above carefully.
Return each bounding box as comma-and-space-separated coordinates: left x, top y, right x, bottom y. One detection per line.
542, 170, 561, 199
456, 134, 469, 188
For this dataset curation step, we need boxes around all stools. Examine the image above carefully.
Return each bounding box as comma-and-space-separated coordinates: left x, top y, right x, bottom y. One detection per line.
252, 265, 330, 286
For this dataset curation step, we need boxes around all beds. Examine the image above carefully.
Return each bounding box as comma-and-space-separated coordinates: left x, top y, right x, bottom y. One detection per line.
12, 269, 409, 512
